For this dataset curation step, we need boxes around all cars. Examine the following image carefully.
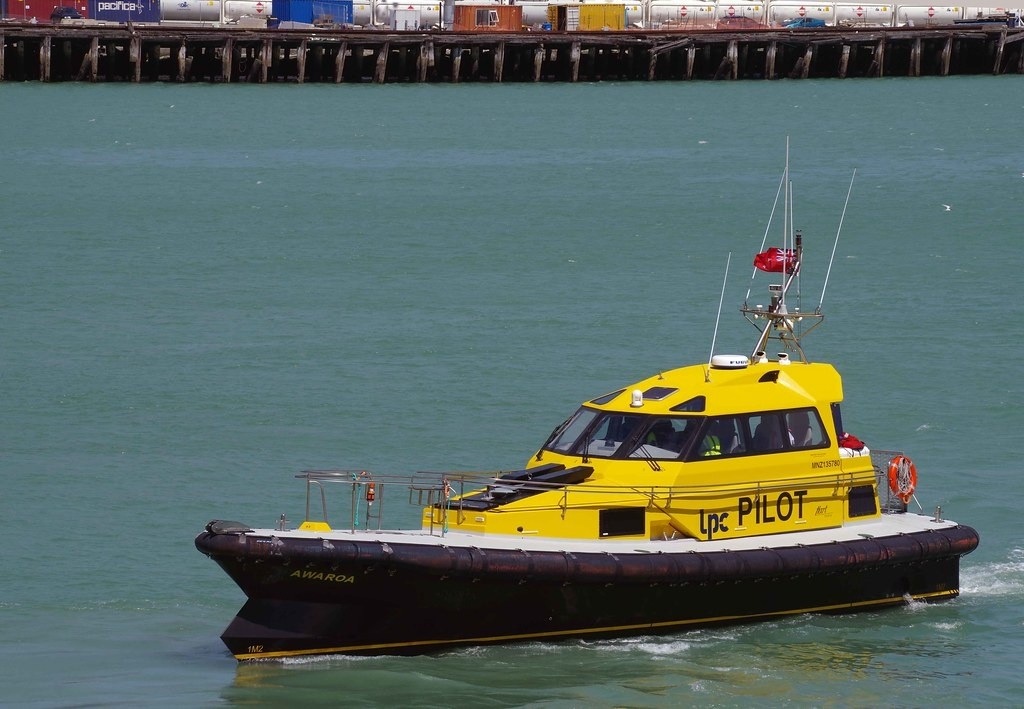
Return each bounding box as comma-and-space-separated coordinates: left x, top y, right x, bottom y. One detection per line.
780, 17, 825, 27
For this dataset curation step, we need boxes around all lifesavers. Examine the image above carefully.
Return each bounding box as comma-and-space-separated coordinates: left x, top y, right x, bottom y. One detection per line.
888, 455, 917, 500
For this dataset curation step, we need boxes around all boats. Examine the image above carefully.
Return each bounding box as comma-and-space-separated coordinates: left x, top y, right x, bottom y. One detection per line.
193, 136, 980, 664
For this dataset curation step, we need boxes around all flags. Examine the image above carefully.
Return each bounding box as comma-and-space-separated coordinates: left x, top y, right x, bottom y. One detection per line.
754, 247, 797, 276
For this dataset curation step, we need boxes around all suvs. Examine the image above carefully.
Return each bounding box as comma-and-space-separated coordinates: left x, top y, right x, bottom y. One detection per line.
717, 16, 769, 29
50, 7, 85, 24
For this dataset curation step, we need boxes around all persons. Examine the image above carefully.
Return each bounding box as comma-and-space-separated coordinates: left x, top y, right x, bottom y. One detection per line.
674, 419, 722, 457
617, 415, 657, 446
787, 410, 813, 446
